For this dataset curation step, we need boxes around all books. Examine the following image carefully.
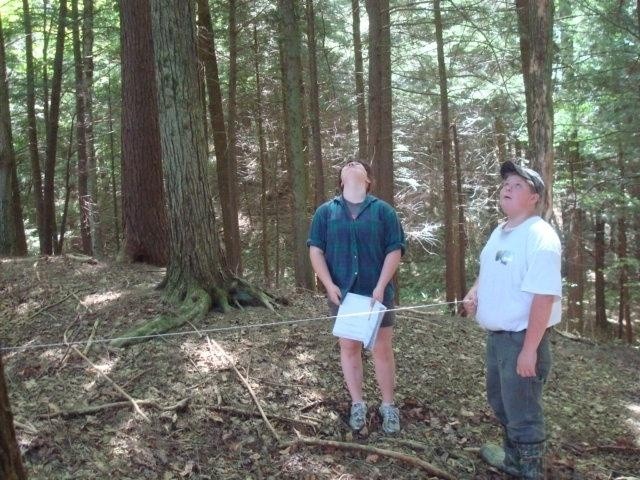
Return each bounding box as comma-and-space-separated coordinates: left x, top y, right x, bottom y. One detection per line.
332, 292, 387, 351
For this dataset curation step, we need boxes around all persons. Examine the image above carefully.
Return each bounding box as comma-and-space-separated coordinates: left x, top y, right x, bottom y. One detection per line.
461, 160, 563, 478
306, 159, 407, 435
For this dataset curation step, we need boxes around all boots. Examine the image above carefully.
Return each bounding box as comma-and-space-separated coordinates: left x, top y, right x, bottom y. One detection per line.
480, 421, 546, 480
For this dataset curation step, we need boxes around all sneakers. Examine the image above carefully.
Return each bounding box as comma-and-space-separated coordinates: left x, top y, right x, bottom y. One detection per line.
379, 403, 400, 434
349, 403, 367, 429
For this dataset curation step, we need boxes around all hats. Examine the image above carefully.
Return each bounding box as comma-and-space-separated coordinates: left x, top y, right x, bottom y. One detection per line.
501, 160, 546, 198
